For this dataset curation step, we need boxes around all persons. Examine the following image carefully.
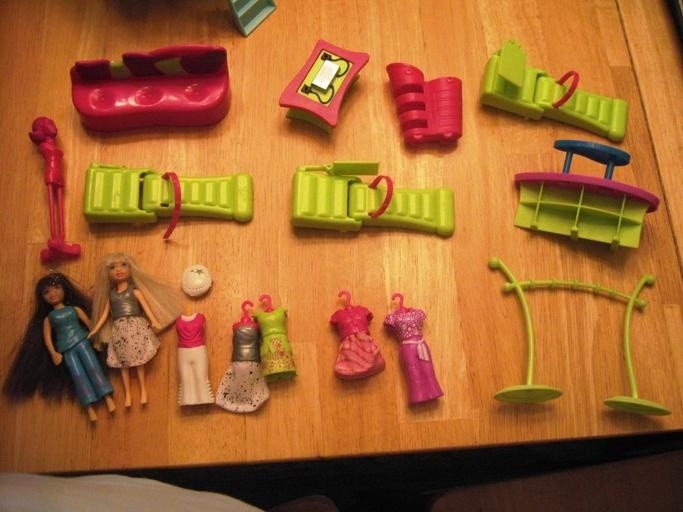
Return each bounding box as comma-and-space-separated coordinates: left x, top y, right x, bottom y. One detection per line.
328, 290, 385, 382
213, 300, 270, 414
0, 272, 117, 421
380, 292, 444, 406
86, 251, 186, 406
28, 115, 81, 263
254, 293, 296, 381
173, 314, 215, 409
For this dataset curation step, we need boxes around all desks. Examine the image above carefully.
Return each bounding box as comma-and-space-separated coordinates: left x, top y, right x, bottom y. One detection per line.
1, 0, 683, 477
